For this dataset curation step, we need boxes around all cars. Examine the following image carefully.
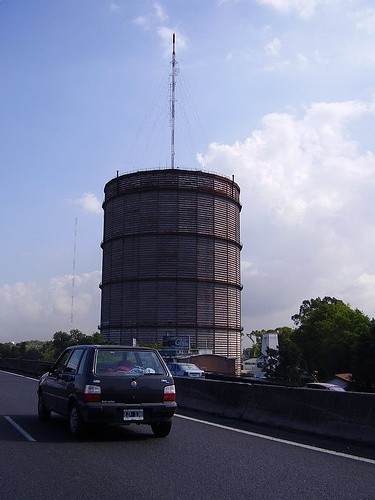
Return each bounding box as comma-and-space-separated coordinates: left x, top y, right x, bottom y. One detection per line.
304, 381, 347, 393
166, 361, 205, 380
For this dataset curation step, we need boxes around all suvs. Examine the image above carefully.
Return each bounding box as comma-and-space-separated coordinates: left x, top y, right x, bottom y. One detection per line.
35, 344, 178, 438
